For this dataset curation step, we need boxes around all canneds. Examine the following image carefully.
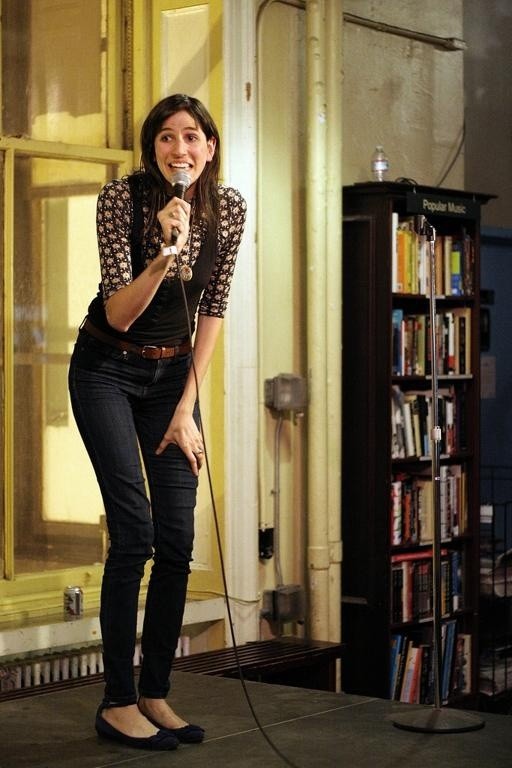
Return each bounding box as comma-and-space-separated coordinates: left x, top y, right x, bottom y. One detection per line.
63, 585, 83, 621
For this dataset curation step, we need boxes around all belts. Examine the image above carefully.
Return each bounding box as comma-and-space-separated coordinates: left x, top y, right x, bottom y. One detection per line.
81, 318, 191, 358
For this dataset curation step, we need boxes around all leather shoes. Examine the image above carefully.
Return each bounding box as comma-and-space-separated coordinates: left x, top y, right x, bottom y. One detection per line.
95, 700, 205, 750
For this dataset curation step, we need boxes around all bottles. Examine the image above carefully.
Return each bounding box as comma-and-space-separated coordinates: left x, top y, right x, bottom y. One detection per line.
371, 145, 389, 181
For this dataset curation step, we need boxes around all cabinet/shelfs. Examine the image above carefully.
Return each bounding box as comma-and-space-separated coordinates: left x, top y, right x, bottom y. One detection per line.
340, 177, 499, 702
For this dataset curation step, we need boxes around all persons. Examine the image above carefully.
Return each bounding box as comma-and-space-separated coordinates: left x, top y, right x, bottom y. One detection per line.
68, 94, 248, 751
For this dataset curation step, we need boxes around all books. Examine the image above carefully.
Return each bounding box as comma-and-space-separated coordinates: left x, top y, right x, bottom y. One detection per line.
479, 644, 512, 697
391, 549, 466, 623
390, 620, 471, 704
391, 385, 457, 459
392, 213, 475, 297
392, 307, 471, 376
391, 465, 466, 546
479, 557, 512, 597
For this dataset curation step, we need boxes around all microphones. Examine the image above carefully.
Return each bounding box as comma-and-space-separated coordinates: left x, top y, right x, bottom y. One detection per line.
172, 171, 191, 245
